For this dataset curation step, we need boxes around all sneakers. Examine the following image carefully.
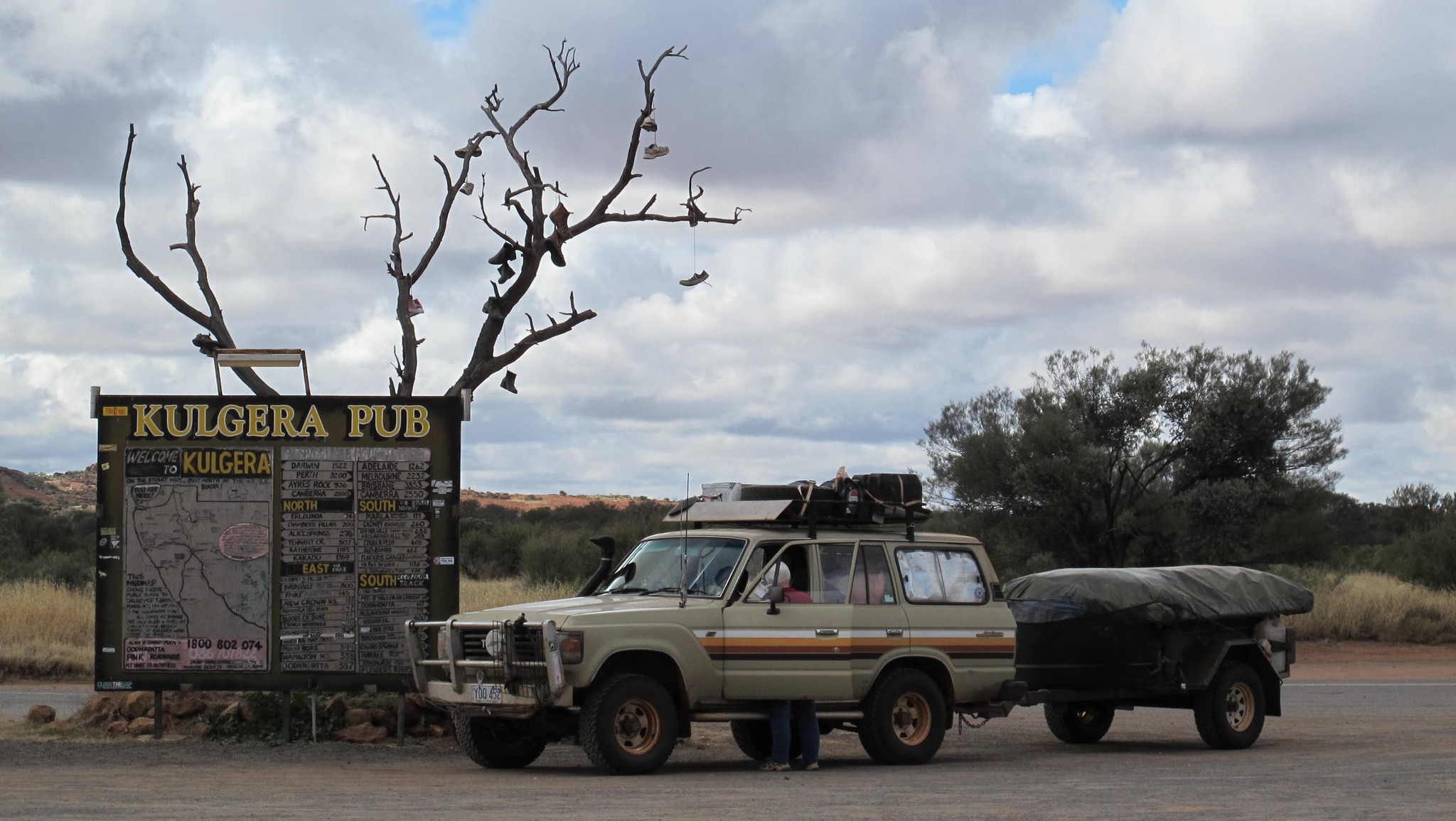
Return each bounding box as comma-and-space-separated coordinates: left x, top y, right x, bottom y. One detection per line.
760, 759, 791, 772
804, 762, 819, 771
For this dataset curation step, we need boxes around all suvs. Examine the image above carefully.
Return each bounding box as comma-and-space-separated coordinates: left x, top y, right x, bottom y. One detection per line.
401, 496, 1019, 776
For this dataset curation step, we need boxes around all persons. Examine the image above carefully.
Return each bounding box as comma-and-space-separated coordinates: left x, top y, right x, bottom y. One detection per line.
758, 562, 821, 773
